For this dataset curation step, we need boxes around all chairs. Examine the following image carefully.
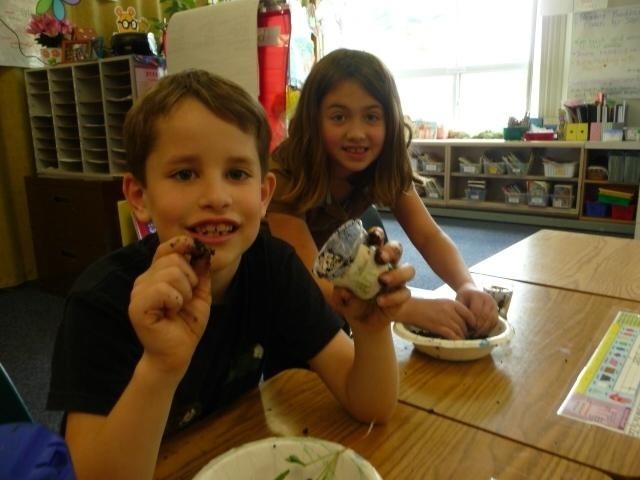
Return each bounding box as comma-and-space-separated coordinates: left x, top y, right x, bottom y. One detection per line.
116, 199, 136, 247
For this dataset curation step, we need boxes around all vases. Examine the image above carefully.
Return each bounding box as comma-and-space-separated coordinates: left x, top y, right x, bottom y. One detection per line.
41, 48, 63, 65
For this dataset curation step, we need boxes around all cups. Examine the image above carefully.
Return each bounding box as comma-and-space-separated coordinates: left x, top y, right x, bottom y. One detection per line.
310, 214, 396, 301
485, 285, 514, 321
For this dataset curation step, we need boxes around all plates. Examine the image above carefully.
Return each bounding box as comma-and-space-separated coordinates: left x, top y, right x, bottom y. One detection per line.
189, 436, 383, 479
392, 314, 517, 365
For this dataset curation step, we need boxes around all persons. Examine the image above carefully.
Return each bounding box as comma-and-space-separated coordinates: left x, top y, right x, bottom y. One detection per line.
46, 69, 415, 480
264, 47, 498, 341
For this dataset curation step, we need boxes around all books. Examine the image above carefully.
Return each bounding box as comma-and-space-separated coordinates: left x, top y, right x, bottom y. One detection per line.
455, 153, 525, 195
423, 177, 442, 193
561, 90, 627, 122
415, 149, 438, 164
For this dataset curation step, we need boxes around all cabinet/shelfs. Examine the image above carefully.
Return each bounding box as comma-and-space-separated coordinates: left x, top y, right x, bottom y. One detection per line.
24, 53, 161, 176
25, 178, 127, 294
371, 139, 638, 233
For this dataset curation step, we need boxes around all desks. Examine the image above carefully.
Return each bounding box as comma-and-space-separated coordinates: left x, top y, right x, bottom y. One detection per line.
155, 228, 639, 479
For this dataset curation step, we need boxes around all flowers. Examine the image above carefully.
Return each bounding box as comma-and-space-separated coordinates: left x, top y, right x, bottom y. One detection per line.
26, 12, 72, 46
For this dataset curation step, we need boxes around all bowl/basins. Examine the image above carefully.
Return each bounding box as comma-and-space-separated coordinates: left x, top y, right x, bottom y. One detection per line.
502, 127, 529, 141
109, 31, 147, 56
